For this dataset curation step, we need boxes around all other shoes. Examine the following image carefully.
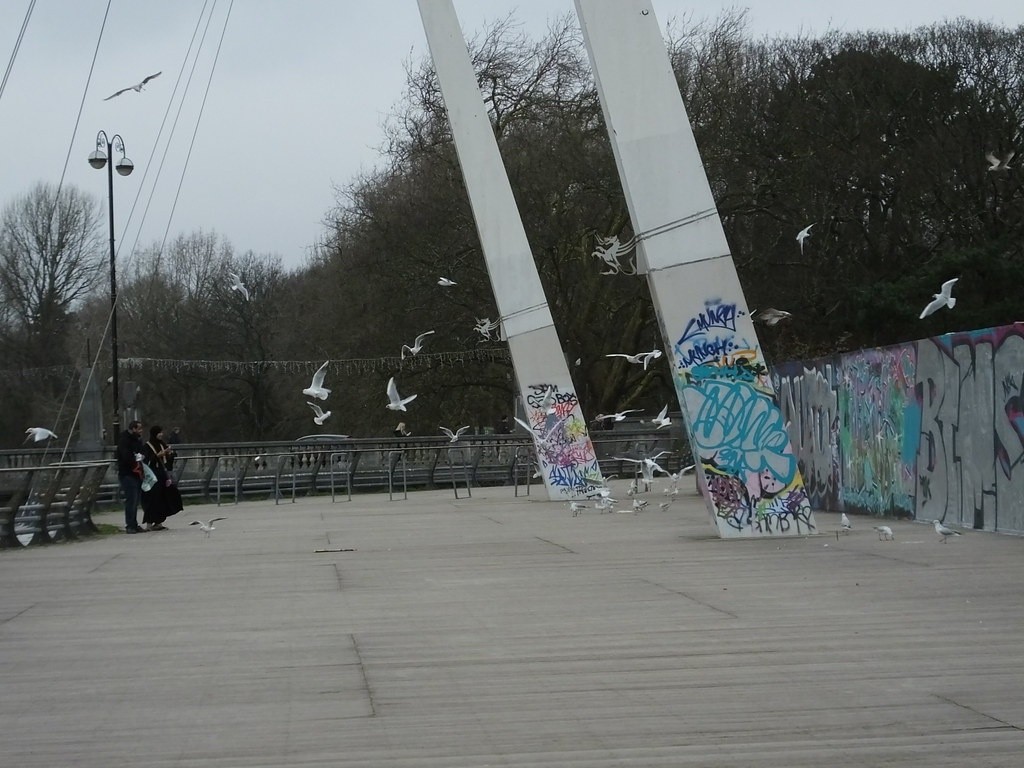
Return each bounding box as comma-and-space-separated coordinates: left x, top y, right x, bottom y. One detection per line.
126, 528, 136, 535
145, 525, 161, 531
154, 524, 169, 530
135, 525, 147, 532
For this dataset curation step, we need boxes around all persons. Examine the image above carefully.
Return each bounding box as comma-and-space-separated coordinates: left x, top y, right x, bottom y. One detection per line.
167, 427, 181, 444
141, 426, 183, 530
590, 414, 614, 431
497, 415, 514, 452
117, 421, 146, 533
394, 422, 410, 462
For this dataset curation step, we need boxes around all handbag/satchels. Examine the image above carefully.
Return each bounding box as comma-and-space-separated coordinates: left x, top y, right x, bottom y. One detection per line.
139, 463, 158, 492
166, 470, 179, 485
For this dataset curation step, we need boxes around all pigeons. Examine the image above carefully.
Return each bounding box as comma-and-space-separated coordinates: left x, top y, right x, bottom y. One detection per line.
919, 277, 959, 319
873, 526, 896, 541
931, 520, 962, 544
984, 151, 1015, 172
796, 223, 815, 256
758, 307, 793, 326
230, 273, 249, 301
841, 513, 853, 536
23, 427, 58, 445
188, 517, 228, 537
103, 71, 162, 102
514, 350, 695, 517
303, 277, 471, 443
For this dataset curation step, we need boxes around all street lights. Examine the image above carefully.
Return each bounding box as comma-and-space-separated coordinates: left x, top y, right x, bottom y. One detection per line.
87, 131, 135, 445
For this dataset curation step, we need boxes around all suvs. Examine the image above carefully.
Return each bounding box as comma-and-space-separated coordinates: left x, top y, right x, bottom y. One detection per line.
254, 434, 357, 469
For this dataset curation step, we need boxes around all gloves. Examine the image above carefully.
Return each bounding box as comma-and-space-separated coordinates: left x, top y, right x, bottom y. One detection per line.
134, 453, 144, 462
